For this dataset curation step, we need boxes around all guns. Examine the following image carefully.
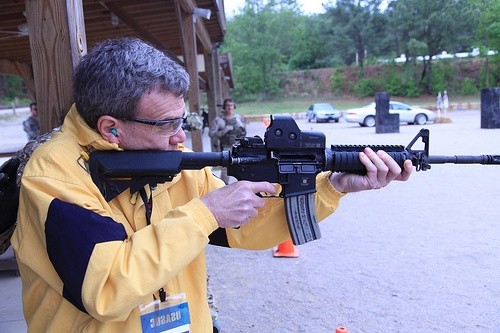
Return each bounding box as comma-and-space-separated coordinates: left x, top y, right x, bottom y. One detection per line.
87, 111, 498, 250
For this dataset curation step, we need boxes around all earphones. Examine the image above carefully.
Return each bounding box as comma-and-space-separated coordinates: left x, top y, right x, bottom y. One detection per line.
111, 129, 116, 136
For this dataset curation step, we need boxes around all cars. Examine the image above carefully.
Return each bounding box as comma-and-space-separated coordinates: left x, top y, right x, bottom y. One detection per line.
305, 103, 340, 123
345, 101, 434, 126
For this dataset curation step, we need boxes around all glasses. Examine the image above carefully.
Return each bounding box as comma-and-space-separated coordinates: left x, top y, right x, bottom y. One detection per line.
126, 109, 189, 137
32, 108, 37, 110
